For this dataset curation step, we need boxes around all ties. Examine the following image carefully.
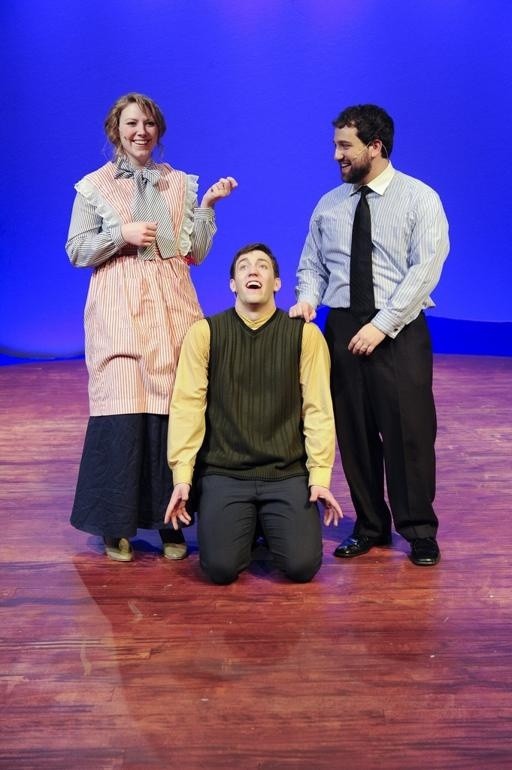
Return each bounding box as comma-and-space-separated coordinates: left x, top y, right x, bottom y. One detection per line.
349, 185, 376, 318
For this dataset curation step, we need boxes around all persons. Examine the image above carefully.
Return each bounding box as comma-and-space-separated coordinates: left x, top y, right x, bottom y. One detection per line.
164, 242, 344, 584
287, 103, 449, 565
65, 90, 239, 562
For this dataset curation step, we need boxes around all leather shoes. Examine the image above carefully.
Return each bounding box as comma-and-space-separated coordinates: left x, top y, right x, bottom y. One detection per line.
334, 532, 392, 558
158, 542, 187, 560
104, 539, 135, 562
409, 537, 441, 565
